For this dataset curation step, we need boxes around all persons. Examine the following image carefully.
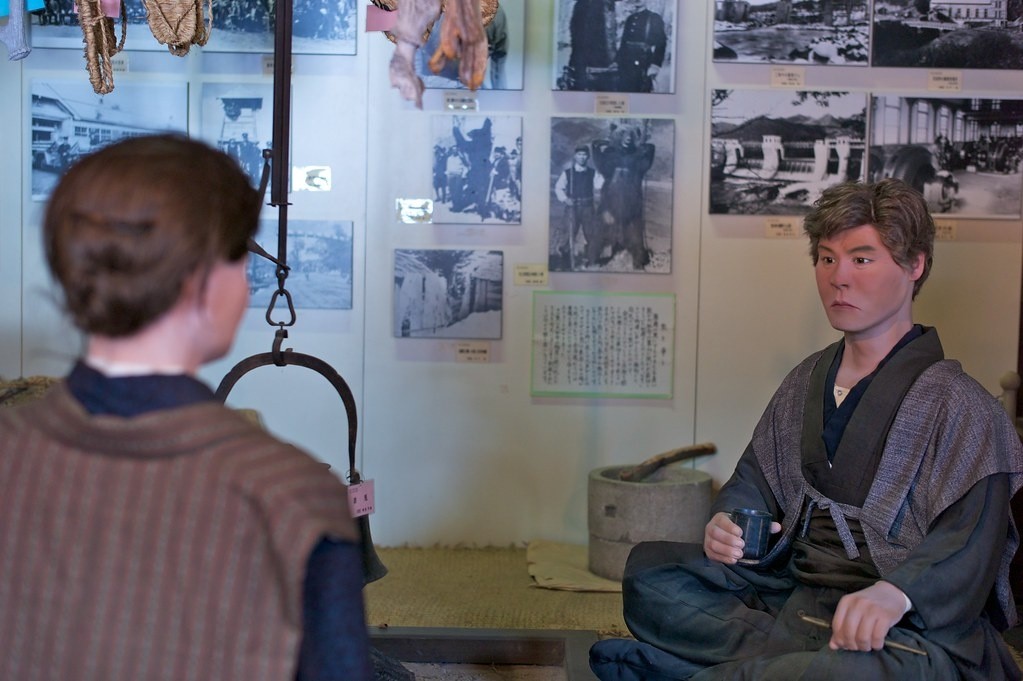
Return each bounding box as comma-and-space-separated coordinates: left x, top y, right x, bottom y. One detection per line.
555, 143, 605, 268
565, 0, 667, 89
33, 0, 352, 44
58, 136, 71, 171
220, 132, 272, 186
0, 130, 419, 681
589, 178, 1023, 681
434, 137, 522, 220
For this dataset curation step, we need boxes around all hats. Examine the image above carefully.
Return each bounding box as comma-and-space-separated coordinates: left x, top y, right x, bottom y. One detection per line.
574, 145, 589, 153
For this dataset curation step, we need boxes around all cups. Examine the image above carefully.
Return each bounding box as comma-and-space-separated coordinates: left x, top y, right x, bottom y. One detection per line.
730, 506, 772, 565
